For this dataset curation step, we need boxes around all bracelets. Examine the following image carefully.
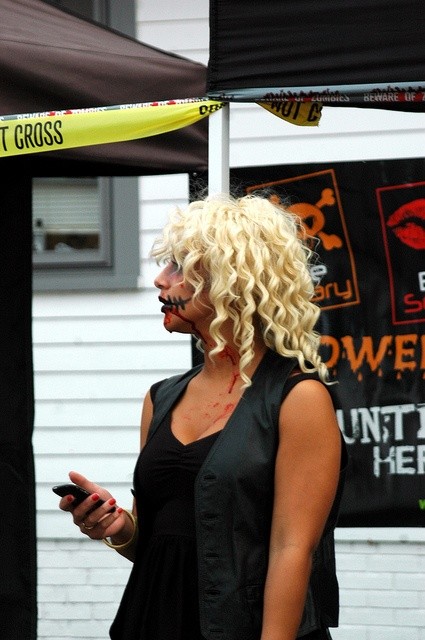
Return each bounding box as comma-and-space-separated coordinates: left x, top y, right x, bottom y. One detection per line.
103, 508, 138, 550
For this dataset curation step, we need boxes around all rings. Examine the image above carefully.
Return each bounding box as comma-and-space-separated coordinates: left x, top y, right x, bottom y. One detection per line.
81, 522, 94, 532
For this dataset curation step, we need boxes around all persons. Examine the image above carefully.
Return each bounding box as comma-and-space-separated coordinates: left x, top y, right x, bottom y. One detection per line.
60, 191, 344, 638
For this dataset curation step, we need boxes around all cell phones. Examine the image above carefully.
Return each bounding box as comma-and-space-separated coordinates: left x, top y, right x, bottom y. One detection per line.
53, 485, 117, 514
